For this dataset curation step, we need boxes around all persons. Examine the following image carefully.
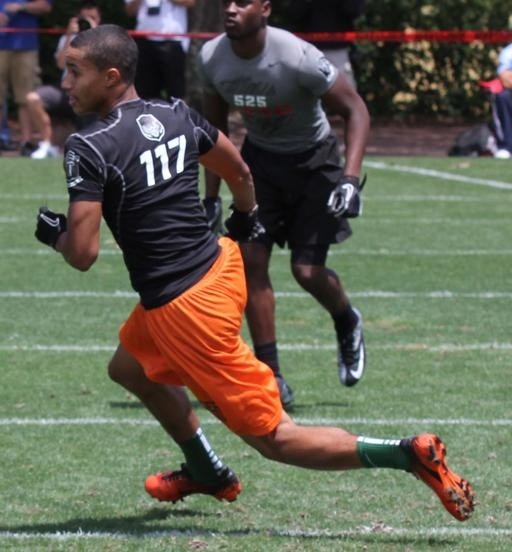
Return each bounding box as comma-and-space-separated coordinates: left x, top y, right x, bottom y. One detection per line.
34, 24, 478, 522
1, 0, 197, 160
490, 42, 512, 160
193, 1, 372, 408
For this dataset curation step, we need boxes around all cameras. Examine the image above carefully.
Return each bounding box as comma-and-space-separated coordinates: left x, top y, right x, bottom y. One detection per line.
78, 15, 91, 32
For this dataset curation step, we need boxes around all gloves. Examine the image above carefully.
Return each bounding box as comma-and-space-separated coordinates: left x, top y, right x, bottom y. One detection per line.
34, 206, 67, 250
203, 196, 227, 239
327, 175, 362, 220
224, 203, 266, 243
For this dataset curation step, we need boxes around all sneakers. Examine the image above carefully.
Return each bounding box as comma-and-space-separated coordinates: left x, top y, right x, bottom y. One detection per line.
399, 434, 475, 521
336, 307, 366, 387
273, 373, 295, 411
20, 141, 59, 159
144, 463, 242, 504
493, 149, 512, 158
